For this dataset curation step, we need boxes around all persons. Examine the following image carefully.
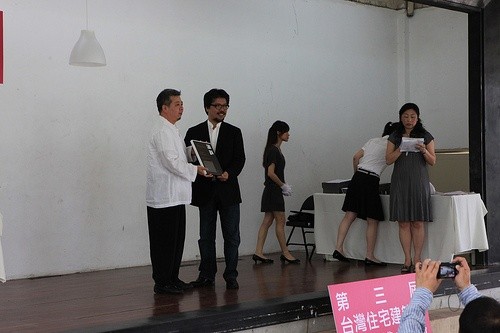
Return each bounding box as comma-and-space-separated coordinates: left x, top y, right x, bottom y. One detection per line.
398, 257, 500, 333
446, 270, 449, 276
333, 121, 399, 264
386, 102, 435, 273
251, 121, 301, 264
183, 89, 247, 288
145, 89, 213, 295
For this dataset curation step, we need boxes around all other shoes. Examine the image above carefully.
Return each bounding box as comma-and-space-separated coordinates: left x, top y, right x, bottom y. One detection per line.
410, 264, 415, 273
189, 277, 215, 287
178, 279, 194, 290
154, 283, 184, 293
226, 279, 239, 289
401, 263, 412, 273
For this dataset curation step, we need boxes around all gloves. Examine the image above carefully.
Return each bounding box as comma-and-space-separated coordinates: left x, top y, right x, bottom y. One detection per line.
281, 184, 293, 196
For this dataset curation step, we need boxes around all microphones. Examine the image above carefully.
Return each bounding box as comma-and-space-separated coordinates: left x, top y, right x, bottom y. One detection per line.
405, 133, 410, 156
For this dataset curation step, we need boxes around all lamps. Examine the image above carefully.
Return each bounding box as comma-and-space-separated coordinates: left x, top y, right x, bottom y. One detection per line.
68, 0, 107, 66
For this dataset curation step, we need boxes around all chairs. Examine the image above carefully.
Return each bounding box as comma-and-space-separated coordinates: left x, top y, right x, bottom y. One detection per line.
285, 195, 315, 262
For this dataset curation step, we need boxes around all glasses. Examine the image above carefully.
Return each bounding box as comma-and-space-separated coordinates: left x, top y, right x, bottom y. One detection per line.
211, 103, 229, 110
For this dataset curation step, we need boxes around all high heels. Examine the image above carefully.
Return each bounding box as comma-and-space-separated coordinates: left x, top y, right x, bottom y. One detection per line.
279, 255, 301, 264
252, 254, 273, 264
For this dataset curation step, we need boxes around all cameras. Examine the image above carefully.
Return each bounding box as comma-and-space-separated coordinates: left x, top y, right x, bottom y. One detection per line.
437, 262, 460, 279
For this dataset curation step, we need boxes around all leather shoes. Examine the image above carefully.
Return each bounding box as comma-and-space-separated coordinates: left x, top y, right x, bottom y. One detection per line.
365, 258, 387, 267
333, 250, 350, 262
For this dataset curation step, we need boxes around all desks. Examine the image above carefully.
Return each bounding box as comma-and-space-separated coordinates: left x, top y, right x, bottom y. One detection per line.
312, 191, 489, 267
